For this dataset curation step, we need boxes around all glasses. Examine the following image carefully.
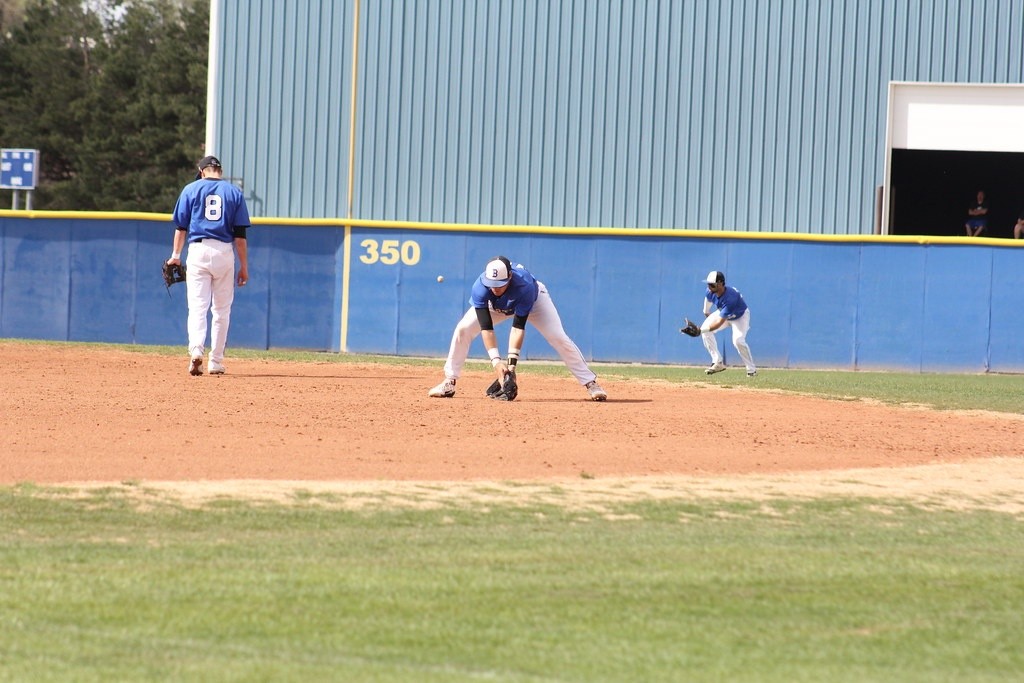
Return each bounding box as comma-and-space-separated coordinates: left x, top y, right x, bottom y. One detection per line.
707, 283, 717, 288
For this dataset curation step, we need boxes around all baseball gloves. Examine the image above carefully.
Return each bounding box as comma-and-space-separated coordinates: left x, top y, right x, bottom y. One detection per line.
162, 258, 186, 299
680, 317, 701, 338
486, 371, 518, 401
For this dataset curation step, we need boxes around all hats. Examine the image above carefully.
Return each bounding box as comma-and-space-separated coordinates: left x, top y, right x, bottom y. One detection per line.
481, 255, 513, 287
701, 270, 726, 284
195, 156, 221, 181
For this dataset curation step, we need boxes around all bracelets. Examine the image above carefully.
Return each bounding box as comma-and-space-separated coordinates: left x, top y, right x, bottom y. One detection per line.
171, 252, 180, 260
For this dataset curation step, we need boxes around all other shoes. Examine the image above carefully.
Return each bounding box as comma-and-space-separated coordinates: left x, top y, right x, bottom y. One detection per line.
747, 371, 757, 378
189, 347, 204, 376
208, 360, 225, 374
704, 362, 726, 375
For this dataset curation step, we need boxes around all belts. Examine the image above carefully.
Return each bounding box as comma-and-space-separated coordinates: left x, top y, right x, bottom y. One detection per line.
193, 238, 203, 243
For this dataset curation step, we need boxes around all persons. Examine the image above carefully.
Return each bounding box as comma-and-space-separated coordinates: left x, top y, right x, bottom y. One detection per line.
1014, 217, 1024, 239
681, 270, 757, 377
428, 255, 607, 402
965, 190, 988, 236
162, 155, 251, 377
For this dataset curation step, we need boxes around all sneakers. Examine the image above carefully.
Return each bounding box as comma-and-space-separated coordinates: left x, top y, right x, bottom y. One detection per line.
429, 379, 456, 397
586, 383, 607, 401
